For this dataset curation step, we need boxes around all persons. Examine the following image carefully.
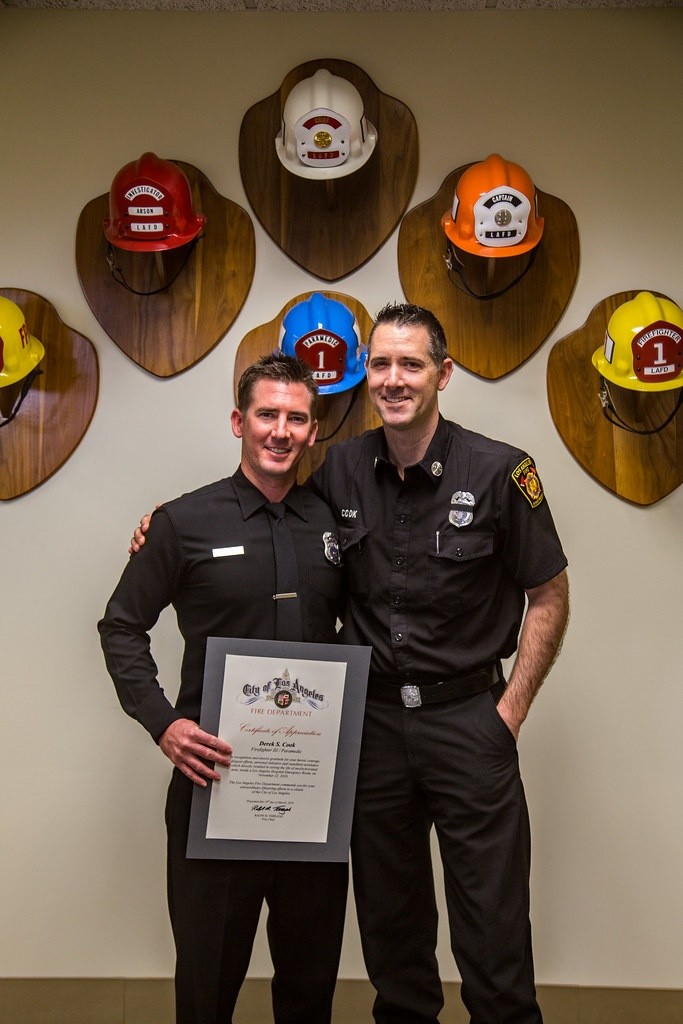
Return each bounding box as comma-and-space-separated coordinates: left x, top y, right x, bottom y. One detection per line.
128, 300, 572, 1024
96, 356, 353, 1024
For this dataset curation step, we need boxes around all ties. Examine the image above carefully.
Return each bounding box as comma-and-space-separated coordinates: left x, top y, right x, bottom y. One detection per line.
266, 504, 305, 641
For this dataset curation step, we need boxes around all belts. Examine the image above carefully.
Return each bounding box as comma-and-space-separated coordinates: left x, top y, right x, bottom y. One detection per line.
366, 664, 500, 710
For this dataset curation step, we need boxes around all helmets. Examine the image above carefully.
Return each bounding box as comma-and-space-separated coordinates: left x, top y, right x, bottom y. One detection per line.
103, 153, 206, 253
272, 292, 369, 396
0, 294, 46, 389
591, 291, 683, 392
441, 154, 545, 258
275, 68, 378, 181
